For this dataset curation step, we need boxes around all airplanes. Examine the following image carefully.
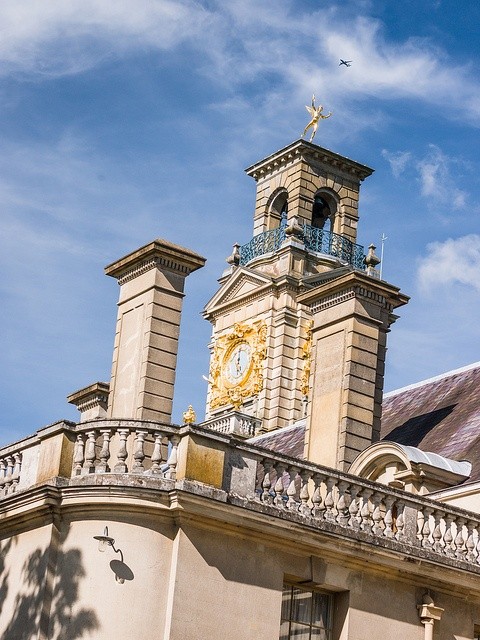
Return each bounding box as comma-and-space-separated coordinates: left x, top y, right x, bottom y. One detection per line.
339, 59, 352, 67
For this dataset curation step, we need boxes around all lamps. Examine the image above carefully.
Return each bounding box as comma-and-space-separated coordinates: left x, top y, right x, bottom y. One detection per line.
92, 525, 114, 553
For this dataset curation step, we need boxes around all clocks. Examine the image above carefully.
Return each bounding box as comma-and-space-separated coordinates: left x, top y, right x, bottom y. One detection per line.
209, 320, 267, 413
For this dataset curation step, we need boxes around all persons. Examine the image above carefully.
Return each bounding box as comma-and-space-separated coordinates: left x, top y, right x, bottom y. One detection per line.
300, 93, 333, 143
